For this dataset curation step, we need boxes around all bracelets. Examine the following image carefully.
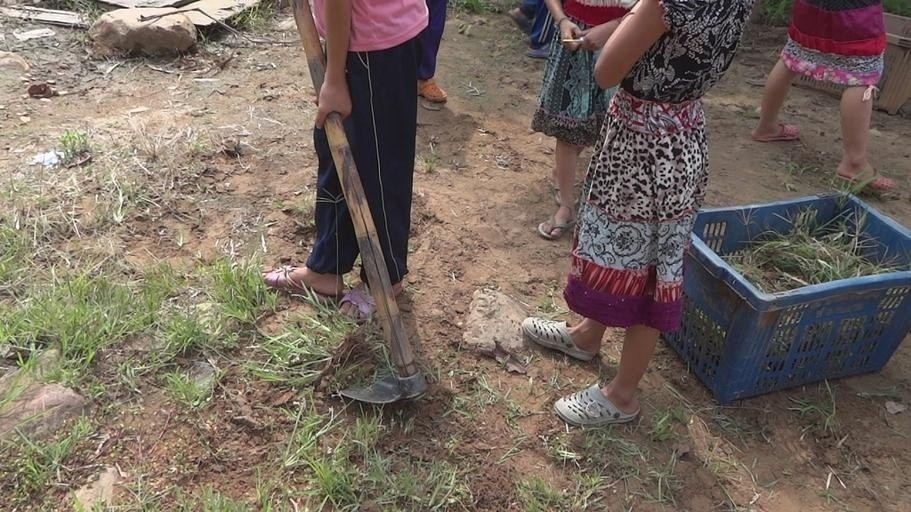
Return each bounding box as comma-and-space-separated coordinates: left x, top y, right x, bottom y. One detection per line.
616, 17, 624, 24
623, 12, 635, 19
556, 16, 571, 27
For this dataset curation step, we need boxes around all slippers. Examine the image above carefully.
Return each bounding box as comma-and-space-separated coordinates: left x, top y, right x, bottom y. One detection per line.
527, 44, 551, 58
755, 123, 801, 141
553, 184, 580, 208
418, 82, 448, 103
554, 383, 640, 428
510, 10, 531, 35
837, 168, 897, 191
539, 214, 577, 240
338, 286, 404, 324
265, 265, 338, 305
523, 316, 593, 361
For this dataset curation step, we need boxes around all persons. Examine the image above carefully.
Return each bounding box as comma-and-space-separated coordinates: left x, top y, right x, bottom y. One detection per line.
521, 0, 750, 425
753, 1, 901, 195
416, 2, 449, 103
259, 1, 428, 328
508, 0, 554, 61
530, 0, 643, 241
